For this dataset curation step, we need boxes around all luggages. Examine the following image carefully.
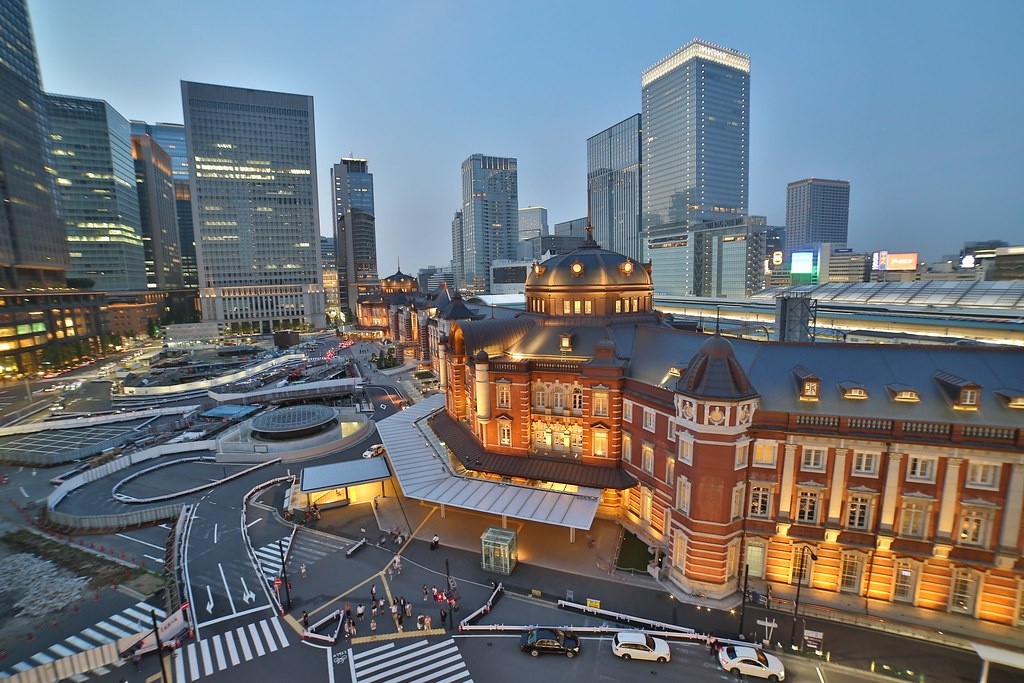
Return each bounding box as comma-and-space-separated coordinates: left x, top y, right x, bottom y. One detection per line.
430, 543, 434, 551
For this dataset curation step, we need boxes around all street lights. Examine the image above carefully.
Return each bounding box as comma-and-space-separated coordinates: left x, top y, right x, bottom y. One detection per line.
790, 546, 818, 647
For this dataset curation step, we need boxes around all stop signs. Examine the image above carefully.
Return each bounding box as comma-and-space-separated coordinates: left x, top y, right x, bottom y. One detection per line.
275, 579, 281, 585
181, 602, 189, 610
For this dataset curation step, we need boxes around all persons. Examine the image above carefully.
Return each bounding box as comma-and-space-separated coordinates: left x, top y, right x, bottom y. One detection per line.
433, 534, 439, 549
380, 527, 408, 546
285, 511, 289, 519
345, 552, 448, 634
303, 611, 308, 626
304, 502, 321, 519
706, 631, 724, 655
301, 565, 306, 578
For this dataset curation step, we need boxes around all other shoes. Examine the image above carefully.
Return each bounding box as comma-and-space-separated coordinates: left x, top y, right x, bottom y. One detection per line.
401, 626, 403, 628
398, 628, 400, 630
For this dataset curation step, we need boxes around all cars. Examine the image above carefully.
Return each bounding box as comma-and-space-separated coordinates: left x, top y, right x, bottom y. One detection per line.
612, 632, 671, 663
362, 445, 384, 458
520, 628, 581, 658
719, 646, 786, 683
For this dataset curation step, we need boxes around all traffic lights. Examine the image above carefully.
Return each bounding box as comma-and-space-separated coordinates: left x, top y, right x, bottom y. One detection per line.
447, 598, 456, 605
444, 592, 454, 600
436, 593, 443, 600
122, 641, 146, 659
